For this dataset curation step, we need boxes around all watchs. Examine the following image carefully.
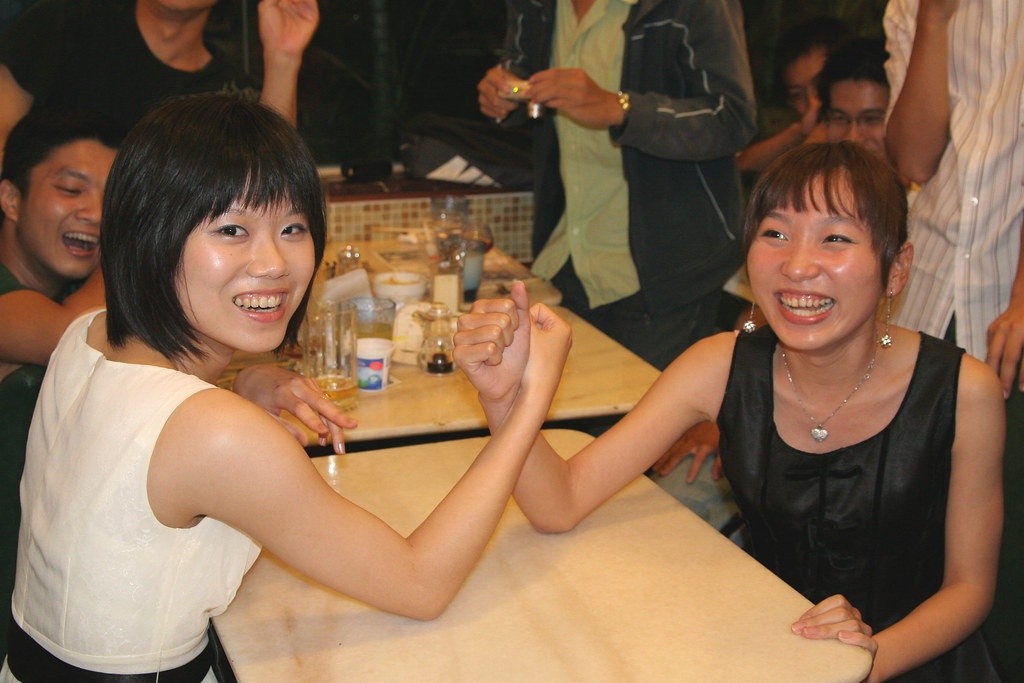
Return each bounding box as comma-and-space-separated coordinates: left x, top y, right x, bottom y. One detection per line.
617, 90, 630, 111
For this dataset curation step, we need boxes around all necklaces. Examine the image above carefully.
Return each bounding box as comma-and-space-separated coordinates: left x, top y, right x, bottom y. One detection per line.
782, 324, 878, 442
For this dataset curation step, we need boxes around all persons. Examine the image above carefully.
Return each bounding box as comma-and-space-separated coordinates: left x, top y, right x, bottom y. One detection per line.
0, 93, 574, 683
0, 106, 129, 671
872, 0, 1024, 683
478, 0, 758, 372
653, 16, 921, 549
452, 140, 1006, 683
0, 0, 319, 169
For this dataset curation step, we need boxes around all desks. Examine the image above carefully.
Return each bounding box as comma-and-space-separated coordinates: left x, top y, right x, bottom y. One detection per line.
211, 242, 873, 683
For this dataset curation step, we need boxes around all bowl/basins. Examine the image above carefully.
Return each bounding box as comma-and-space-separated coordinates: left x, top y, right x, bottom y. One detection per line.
370, 269, 428, 304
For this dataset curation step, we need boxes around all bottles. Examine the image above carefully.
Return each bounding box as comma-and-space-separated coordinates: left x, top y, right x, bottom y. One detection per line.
418, 306, 461, 376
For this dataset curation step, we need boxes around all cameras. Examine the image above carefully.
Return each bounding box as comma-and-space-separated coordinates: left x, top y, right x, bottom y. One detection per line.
499, 80, 544, 120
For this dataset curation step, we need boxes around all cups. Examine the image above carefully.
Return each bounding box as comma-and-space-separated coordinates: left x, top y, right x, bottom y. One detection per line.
354, 337, 396, 391
298, 300, 363, 410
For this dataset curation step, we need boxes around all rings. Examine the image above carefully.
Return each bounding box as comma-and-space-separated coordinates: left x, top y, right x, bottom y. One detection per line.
321, 392, 331, 400
